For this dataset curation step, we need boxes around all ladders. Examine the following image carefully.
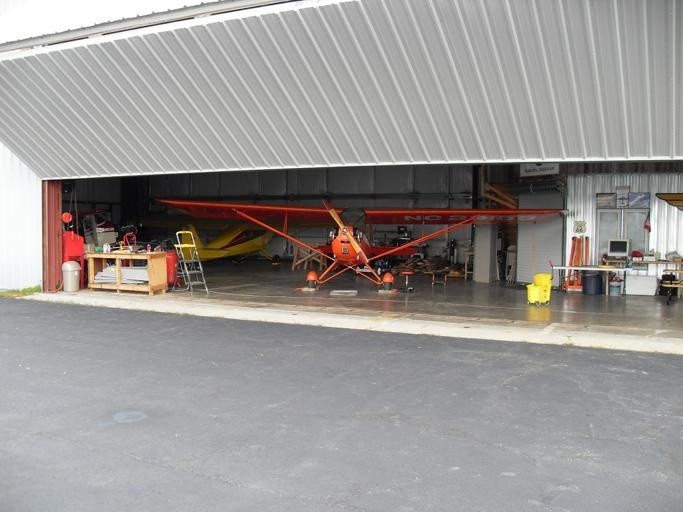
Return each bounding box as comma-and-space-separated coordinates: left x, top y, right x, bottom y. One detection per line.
169, 229, 209, 298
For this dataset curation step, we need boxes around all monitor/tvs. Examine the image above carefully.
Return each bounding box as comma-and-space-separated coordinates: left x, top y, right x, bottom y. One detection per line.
607, 238, 629, 257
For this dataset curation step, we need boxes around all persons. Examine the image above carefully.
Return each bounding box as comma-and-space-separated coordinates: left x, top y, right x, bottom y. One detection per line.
230, 232, 248, 266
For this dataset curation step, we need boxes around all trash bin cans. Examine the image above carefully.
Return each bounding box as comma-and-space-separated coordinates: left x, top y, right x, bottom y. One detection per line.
580, 269, 602, 295
61, 259, 83, 294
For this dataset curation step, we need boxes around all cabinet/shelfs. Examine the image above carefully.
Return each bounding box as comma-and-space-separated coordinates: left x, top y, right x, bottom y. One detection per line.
85, 252, 167, 296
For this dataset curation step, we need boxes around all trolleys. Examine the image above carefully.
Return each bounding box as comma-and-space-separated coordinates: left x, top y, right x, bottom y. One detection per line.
526, 265, 553, 308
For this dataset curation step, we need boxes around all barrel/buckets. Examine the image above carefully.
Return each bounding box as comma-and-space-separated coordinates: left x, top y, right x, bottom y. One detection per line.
581, 264, 603, 295
165, 249, 180, 286
609, 282, 621, 296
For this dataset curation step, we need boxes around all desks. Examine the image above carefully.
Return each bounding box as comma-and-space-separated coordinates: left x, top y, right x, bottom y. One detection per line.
550, 265, 632, 296
